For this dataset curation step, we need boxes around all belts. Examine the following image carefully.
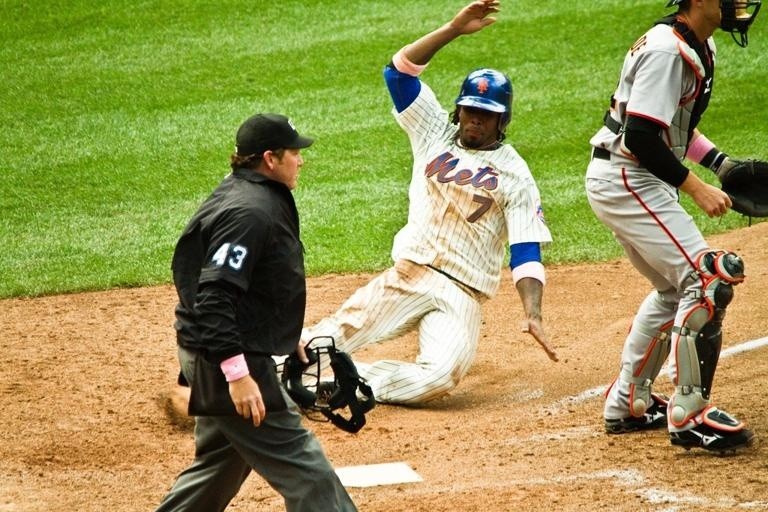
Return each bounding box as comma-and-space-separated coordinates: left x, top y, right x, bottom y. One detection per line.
592, 145, 611, 163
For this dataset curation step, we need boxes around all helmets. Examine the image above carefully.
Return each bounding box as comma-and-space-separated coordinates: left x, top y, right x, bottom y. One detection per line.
454, 67, 513, 115
273, 336, 376, 435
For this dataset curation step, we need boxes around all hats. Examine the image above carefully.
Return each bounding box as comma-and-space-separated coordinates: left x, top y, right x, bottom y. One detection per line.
235, 113, 315, 157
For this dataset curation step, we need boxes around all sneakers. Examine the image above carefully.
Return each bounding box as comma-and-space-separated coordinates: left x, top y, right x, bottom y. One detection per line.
604, 399, 667, 434
669, 420, 756, 452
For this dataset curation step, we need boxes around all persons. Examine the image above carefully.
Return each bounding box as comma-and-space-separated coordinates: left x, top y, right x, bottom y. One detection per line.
161, 0, 559, 422
585, 0, 768, 450
155, 112, 364, 512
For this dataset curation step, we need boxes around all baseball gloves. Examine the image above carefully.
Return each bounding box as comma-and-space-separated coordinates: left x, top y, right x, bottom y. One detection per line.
721, 160, 767, 217
